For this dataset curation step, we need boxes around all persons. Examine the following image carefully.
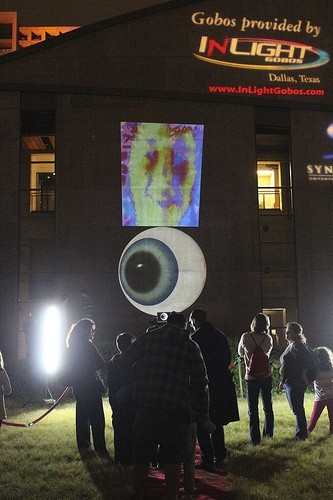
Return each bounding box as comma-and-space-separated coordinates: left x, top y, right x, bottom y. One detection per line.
146, 325, 196, 500
0, 352, 12, 426
67, 317, 110, 455
109, 332, 136, 465
121, 121, 199, 227
115, 313, 209, 500
237, 313, 274, 445
189, 309, 240, 470
307, 346, 333, 435
278, 322, 312, 440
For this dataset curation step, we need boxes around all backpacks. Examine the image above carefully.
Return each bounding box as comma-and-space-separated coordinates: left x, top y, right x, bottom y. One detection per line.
247, 332, 271, 380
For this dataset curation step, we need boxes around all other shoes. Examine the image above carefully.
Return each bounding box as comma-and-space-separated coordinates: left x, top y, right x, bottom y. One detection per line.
213, 460, 225, 470
196, 462, 207, 469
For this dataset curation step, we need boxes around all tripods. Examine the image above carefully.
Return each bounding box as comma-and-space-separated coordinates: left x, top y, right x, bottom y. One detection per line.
23, 378, 55, 408
